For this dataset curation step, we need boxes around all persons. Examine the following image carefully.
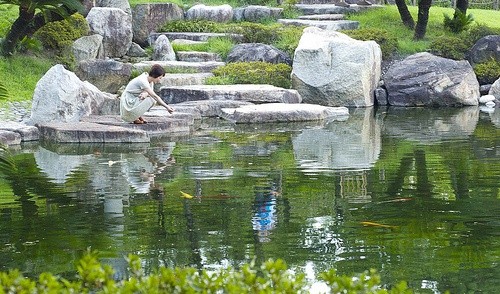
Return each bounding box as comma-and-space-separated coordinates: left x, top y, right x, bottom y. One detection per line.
124, 147, 169, 200
120, 64, 176, 124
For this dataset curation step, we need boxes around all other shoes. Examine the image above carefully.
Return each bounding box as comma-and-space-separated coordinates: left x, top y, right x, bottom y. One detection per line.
130, 120, 147, 124
